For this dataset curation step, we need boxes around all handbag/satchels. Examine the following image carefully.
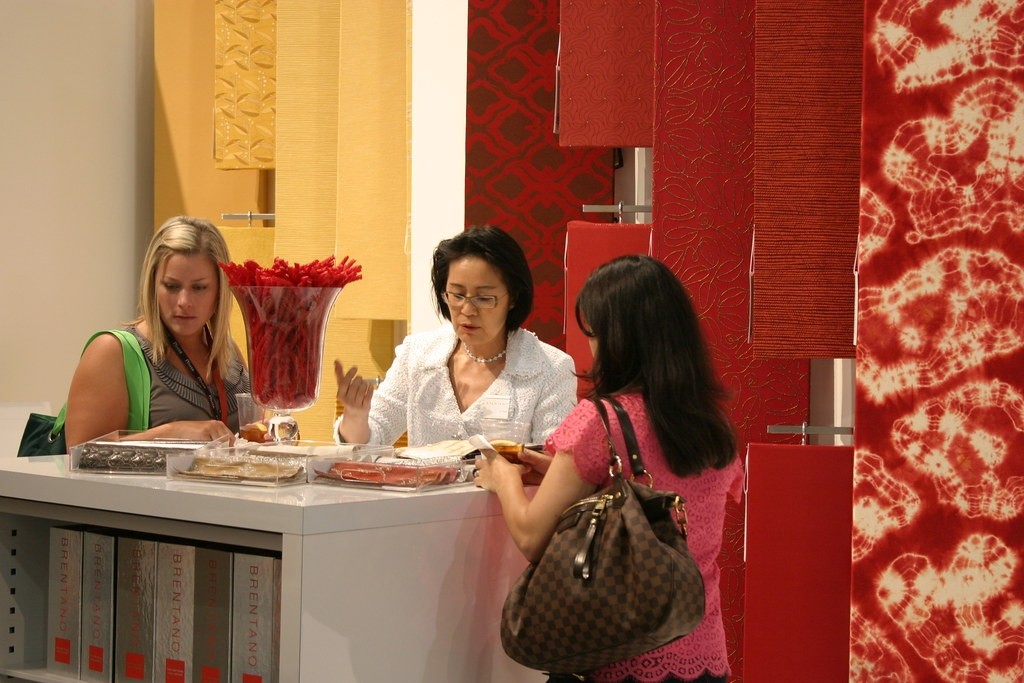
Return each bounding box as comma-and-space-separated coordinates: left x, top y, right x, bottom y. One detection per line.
500, 397, 706, 673
16, 330, 151, 457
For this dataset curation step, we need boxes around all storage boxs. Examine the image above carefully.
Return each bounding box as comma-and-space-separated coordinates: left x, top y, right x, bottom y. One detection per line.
46, 524, 282, 683
68, 430, 479, 494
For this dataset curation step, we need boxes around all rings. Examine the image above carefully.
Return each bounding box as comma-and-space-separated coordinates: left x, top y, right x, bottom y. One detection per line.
473, 468, 479, 478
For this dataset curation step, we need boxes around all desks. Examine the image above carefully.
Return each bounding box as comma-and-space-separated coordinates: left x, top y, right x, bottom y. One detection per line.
0, 452, 550, 683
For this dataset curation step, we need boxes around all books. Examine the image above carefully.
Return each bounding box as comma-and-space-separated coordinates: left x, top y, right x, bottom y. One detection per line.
47, 523, 282, 683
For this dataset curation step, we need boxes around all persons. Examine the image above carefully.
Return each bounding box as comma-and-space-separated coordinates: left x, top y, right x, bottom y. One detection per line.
472, 253, 745, 683
63, 216, 300, 459
332, 223, 578, 455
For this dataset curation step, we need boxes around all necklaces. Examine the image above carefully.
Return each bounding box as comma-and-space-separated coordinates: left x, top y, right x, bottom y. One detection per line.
463, 343, 507, 364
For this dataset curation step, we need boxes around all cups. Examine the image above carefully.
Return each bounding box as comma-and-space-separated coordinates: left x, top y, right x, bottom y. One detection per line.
236, 393, 270, 444
479, 420, 531, 486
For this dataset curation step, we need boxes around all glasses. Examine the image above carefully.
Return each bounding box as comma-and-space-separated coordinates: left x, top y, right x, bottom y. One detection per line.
440, 291, 508, 309
